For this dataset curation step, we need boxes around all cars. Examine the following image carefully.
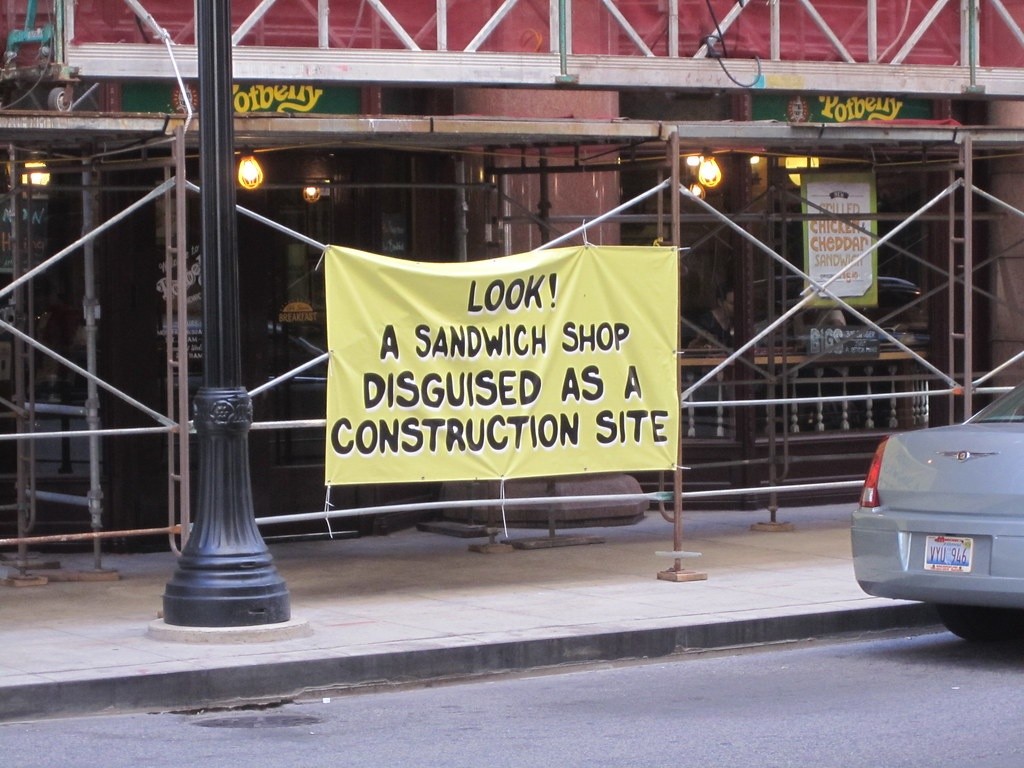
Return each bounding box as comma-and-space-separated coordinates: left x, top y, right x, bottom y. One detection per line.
848, 378, 1024, 644
0, 313, 442, 549
688, 273, 930, 427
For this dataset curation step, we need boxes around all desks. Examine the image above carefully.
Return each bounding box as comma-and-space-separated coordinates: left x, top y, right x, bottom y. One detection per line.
679, 350, 931, 437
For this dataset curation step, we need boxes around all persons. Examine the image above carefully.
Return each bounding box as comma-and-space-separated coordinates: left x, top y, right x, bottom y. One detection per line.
793, 309, 846, 346
690, 281, 735, 348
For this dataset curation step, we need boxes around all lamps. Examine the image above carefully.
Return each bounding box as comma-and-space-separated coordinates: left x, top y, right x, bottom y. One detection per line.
698, 147, 721, 187
689, 176, 706, 202
238, 144, 264, 193
